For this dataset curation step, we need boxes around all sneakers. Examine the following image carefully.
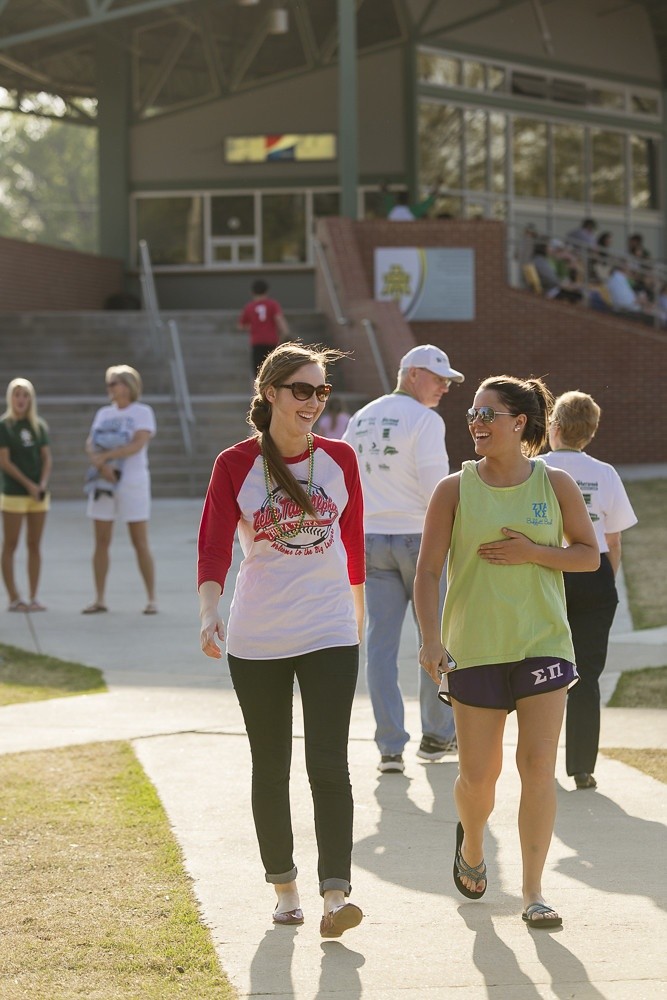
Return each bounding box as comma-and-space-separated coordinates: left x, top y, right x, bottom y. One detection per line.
376, 755, 405, 772
416, 736, 458, 759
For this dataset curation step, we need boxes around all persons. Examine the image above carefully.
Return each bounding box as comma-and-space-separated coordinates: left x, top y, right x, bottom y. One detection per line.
341, 345, 467, 773
81, 363, 160, 615
529, 217, 661, 319
526, 390, 639, 789
196, 342, 368, 938
239, 279, 292, 381
411, 372, 603, 929
1, 377, 52, 613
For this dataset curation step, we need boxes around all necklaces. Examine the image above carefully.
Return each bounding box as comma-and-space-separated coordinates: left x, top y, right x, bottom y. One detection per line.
260, 433, 315, 539
392, 390, 411, 396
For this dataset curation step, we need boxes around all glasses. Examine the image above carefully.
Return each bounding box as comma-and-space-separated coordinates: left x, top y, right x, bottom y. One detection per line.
465, 406, 521, 421
107, 382, 122, 387
278, 382, 333, 402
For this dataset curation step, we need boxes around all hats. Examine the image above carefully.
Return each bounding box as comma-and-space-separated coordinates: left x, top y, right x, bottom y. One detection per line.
400, 344, 464, 383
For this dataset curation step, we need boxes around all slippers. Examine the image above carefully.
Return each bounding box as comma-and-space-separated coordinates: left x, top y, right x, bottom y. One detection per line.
522, 903, 562, 926
453, 821, 488, 899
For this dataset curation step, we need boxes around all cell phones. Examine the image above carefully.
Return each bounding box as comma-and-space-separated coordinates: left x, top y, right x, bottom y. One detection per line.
440, 649, 457, 673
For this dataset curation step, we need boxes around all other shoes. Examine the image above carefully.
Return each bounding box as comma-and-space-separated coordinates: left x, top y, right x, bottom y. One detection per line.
143, 605, 156, 614
82, 605, 106, 613
575, 772, 596, 786
29, 601, 45, 611
9, 600, 29, 612
320, 903, 363, 938
273, 902, 304, 924
